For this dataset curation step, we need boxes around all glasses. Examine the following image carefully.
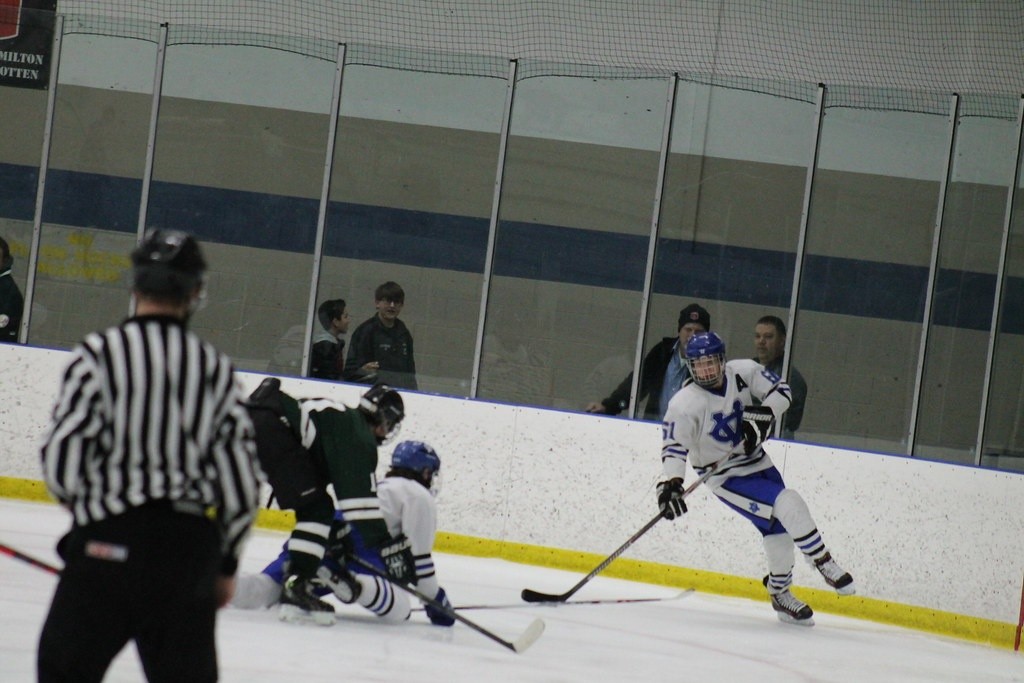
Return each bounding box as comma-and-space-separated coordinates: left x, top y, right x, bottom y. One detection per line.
378, 298, 403, 308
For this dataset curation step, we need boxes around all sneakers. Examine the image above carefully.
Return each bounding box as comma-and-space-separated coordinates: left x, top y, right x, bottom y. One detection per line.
814, 548, 856, 596
314, 561, 362, 604
276, 577, 337, 625
762, 576, 815, 626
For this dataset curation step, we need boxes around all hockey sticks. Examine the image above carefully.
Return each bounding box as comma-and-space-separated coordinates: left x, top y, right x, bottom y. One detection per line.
410, 584, 696, 613
0, 543, 63, 577
521, 437, 746, 604
333, 544, 546, 656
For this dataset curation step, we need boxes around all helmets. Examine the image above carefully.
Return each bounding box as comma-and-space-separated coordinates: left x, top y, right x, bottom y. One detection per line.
129, 227, 208, 287
388, 440, 442, 497
357, 385, 406, 440
686, 331, 727, 390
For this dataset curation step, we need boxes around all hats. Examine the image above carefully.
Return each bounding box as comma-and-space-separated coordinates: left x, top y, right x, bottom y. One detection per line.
678, 303, 712, 332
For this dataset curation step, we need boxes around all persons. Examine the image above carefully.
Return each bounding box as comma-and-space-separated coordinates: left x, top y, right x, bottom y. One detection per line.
0, 236, 23, 343
228, 377, 458, 626
657, 332, 853, 620
584, 303, 808, 440
309, 281, 418, 390
39, 229, 267, 683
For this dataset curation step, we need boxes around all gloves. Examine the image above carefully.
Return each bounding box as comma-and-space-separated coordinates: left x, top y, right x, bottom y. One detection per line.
656, 479, 688, 521
424, 589, 456, 626
732, 406, 776, 457
